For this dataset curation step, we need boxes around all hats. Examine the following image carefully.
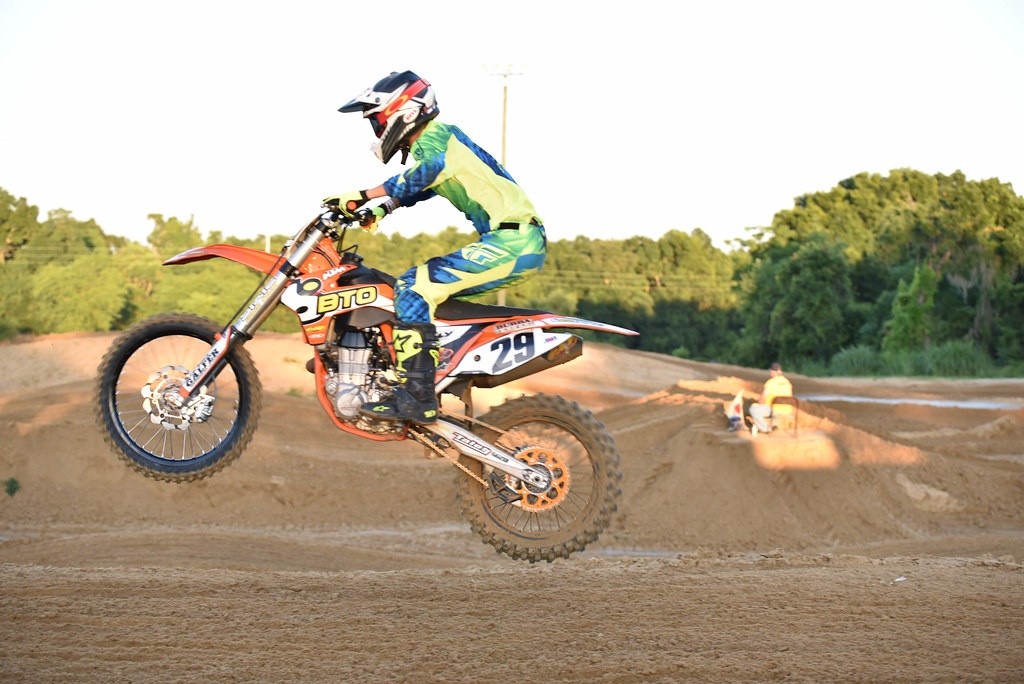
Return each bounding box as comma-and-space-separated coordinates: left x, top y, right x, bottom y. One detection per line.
768, 362, 783, 373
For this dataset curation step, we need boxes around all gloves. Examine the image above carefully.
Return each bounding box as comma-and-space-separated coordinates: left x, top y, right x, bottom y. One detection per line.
353, 195, 395, 224
320, 190, 368, 221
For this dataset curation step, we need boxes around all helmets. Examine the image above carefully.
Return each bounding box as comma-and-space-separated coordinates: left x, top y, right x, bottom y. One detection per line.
337, 70, 440, 165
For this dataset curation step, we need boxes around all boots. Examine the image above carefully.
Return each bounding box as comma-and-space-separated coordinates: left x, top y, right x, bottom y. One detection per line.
306, 357, 317, 373
358, 323, 440, 426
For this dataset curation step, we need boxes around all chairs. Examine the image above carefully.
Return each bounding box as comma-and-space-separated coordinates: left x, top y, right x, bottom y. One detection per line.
764, 397, 799, 434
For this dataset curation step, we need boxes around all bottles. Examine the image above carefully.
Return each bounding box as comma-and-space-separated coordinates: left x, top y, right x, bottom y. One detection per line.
751, 424, 757, 437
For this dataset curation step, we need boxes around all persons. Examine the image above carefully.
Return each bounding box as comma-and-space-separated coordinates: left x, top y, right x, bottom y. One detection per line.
319, 71, 547, 425
749, 363, 792, 433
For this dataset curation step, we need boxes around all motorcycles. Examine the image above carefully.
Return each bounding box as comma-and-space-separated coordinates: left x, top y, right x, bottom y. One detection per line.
91, 198, 623, 564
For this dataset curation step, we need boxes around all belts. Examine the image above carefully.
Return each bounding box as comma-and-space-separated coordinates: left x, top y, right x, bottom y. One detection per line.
499, 218, 539, 229
